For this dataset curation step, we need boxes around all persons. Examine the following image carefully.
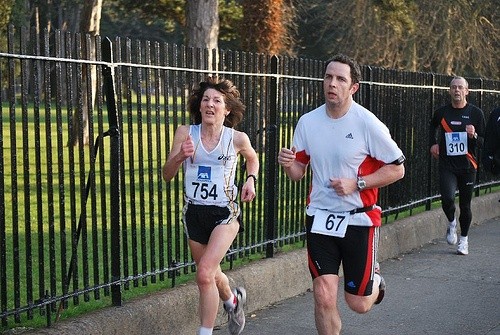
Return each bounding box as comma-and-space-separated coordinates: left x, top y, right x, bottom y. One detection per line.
163, 78, 259, 335
429, 76, 486, 255
278, 56, 405, 334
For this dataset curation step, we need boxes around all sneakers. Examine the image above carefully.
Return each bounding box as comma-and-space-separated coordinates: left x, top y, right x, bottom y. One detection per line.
222, 287, 246, 335
446, 217, 458, 245
457, 236, 468, 254
374, 262, 386, 305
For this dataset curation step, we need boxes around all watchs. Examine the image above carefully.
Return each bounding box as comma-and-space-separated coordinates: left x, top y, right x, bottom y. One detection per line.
356, 176, 366, 191
246, 175, 256, 183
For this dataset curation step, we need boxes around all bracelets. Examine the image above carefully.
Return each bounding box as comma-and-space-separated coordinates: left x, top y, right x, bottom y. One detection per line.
474, 133, 477, 139
